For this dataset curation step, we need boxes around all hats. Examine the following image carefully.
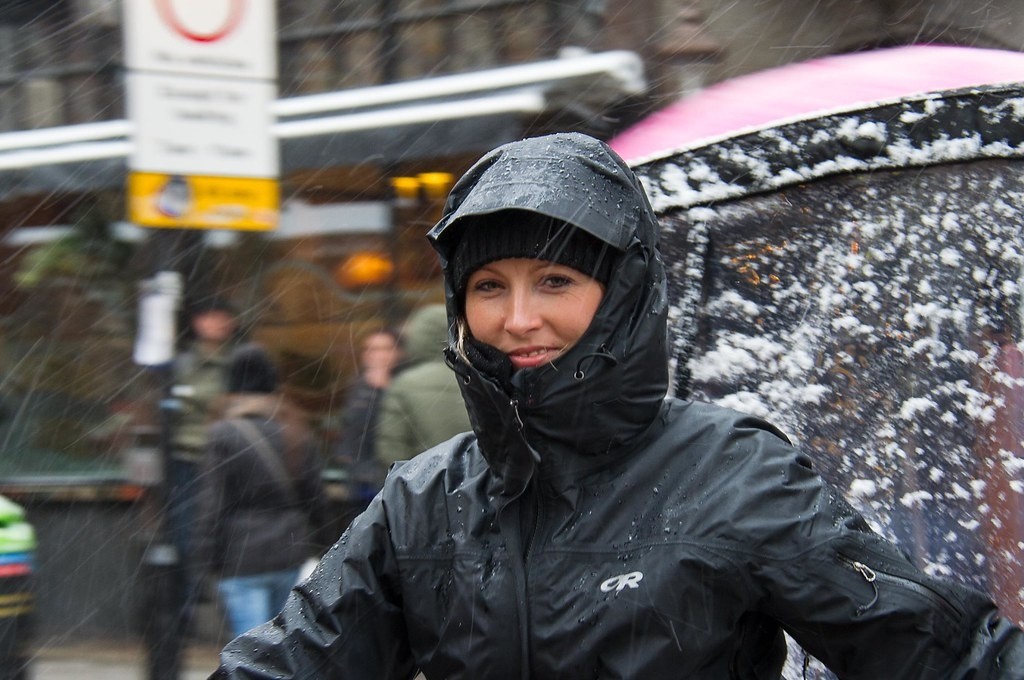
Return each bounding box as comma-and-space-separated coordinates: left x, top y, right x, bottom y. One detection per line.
225, 347, 278, 393
452, 211, 615, 302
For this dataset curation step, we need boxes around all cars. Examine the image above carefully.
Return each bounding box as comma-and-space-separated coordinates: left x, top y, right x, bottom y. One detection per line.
607, 35, 1024, 679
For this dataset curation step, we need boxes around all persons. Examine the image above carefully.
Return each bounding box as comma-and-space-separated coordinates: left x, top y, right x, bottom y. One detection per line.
186, 344, 338, 639
164, 294, 246, 590
206, 134, 1023, 680
339, 331, 405, 515
378, 300, 474, 475
